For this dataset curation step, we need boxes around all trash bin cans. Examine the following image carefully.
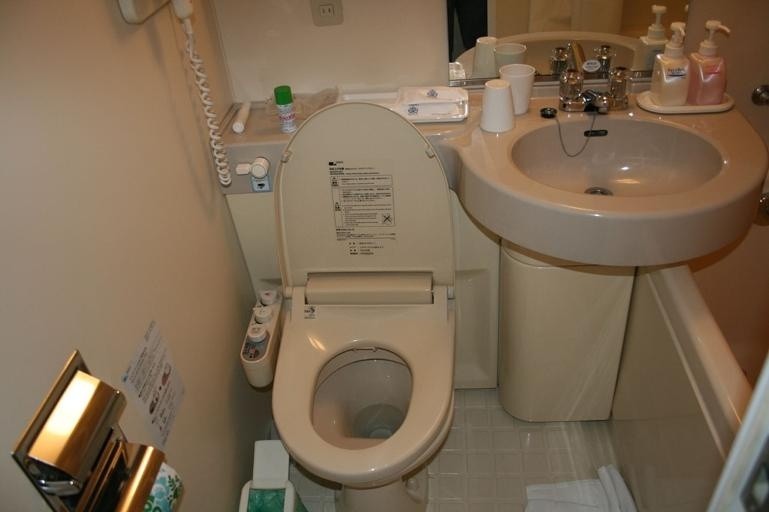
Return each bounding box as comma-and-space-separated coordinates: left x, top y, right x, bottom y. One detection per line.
238, 439, 313, 512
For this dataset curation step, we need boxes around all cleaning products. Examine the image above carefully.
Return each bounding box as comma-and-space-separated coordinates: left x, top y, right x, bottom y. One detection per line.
689, 19, 731, 105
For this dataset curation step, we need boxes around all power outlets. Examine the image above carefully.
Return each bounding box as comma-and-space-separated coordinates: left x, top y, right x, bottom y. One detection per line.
310, 0, 343, 27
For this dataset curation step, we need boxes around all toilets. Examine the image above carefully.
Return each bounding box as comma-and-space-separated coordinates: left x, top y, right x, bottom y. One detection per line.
243, 103, 459, 512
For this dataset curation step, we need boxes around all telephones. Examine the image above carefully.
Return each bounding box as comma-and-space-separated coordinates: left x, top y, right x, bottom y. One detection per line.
168, 0, 233, 187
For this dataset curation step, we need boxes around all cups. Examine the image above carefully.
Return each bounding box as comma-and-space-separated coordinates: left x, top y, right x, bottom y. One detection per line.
480, 79, 516, 133
472, 37, 497, 82
496, 43, 527, 66
499, 63, 535, 115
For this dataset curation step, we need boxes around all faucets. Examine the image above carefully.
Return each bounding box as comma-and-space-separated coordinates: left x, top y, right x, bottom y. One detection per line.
584, 88, 613, 115
562, 38, 590, 71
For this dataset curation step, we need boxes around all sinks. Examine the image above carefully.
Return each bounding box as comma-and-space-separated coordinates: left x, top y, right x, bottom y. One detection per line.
463, 114, 767, 265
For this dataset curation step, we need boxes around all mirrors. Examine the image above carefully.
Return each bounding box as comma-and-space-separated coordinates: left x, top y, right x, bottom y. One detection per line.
447, 0, 691, 87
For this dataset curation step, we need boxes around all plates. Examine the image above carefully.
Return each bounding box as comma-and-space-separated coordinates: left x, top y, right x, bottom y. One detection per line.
636, 91, 734, 114
